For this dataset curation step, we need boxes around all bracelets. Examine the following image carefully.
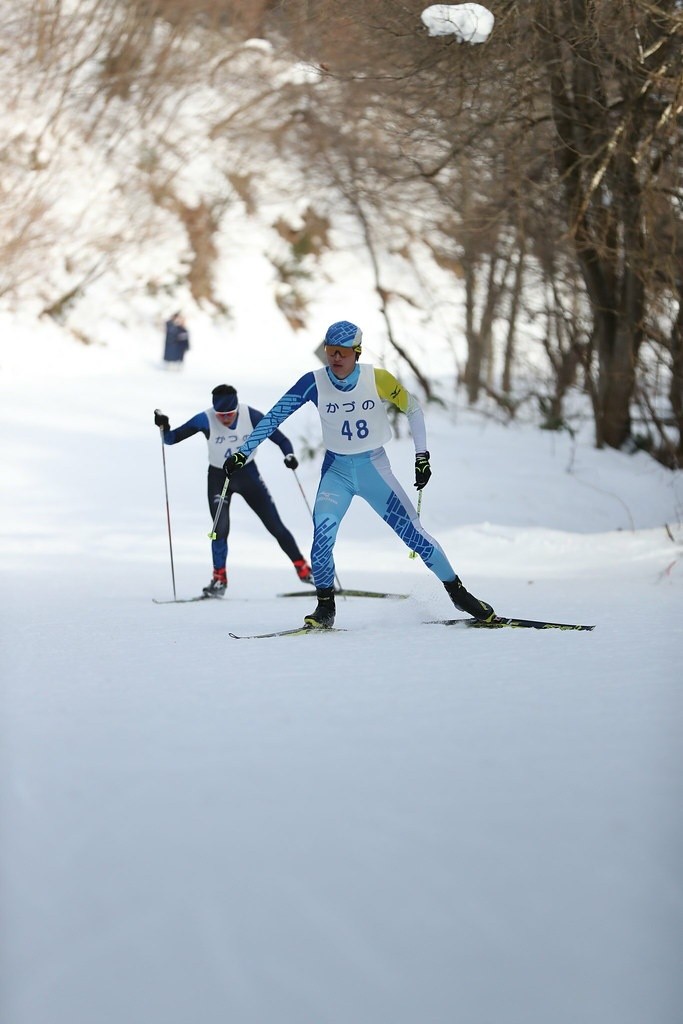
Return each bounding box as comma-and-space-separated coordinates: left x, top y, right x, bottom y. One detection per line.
416, 450, 430, 460
285, 453, 294, 459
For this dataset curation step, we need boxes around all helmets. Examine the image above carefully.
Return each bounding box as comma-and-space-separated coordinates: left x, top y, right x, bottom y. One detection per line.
323, 320, 363, 347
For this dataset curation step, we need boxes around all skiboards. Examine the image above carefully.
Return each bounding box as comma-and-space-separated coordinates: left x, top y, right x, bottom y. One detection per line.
227, 616, 599, 641
148, 587, 413, 606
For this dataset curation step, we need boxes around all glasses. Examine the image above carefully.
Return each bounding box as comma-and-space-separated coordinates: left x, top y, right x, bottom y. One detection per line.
216, 411, 234, 418
324, 345, 354, 357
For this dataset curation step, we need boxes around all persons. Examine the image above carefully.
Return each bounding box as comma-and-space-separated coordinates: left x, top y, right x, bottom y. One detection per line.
223, 321, 497, 628
154, 384, 314, 594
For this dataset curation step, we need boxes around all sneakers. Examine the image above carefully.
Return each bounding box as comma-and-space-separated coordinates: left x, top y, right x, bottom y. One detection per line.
303, 586, 336, 628
442, 575, 498, 624
294, 566, 315, 586
202, 571, 228, 598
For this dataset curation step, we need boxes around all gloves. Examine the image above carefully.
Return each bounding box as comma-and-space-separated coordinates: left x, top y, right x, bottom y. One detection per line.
413, 451, 432, 491
155, 415, 170, 432
223, 451, 248, 480
284, 453, 298, 469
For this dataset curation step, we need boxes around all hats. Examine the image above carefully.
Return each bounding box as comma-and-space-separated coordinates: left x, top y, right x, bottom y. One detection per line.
211, 384, 238, 412
